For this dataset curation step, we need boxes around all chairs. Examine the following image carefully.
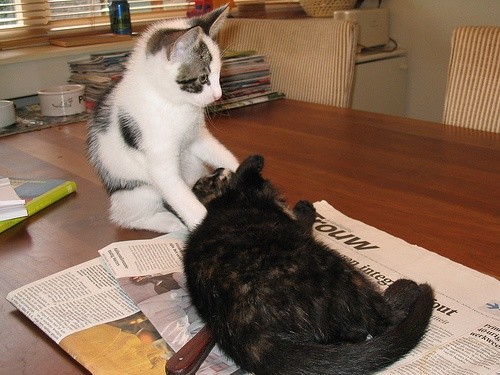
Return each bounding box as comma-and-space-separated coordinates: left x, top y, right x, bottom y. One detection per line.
219, 16, 358, 108
442, 25, 500, 126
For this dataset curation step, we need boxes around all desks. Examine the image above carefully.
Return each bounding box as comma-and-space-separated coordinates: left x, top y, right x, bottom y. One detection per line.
0, 100, 500, 375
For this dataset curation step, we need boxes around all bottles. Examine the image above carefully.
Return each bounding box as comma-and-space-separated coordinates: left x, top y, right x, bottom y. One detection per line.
109, 0, 132, 35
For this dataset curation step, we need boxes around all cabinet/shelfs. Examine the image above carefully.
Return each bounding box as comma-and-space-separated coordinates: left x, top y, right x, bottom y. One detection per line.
352, 46, 408, 114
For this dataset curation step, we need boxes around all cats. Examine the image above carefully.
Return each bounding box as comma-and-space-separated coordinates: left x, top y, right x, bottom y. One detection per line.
178, 151, 437, 375
79, 0, 242, 237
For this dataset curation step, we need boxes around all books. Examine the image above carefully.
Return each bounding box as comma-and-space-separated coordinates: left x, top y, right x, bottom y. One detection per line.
67, 50, 131, 113
0, 176, 76, 234
206, 47, 287, 114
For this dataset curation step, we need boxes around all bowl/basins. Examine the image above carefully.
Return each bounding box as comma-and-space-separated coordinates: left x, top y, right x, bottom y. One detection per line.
0, 100, 16, 128
37, 84, 87, 117
